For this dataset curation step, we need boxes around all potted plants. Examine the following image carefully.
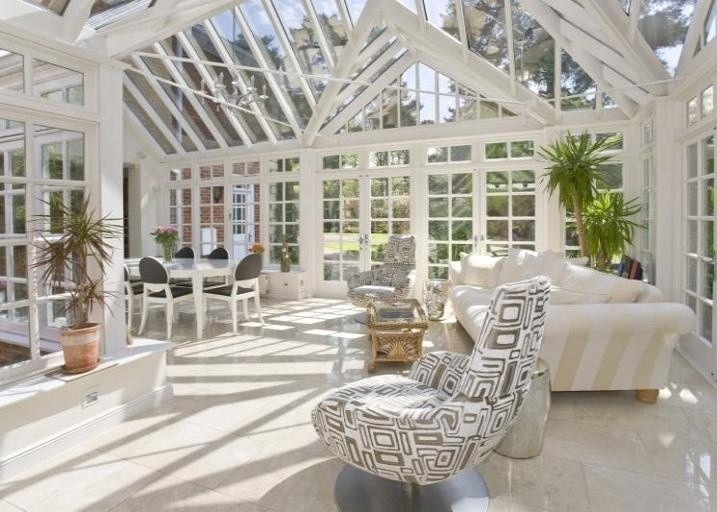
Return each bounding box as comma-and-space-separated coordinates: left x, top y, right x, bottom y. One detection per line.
21, 185, 123, 377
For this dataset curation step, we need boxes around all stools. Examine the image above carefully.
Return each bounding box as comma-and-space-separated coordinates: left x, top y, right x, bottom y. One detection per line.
495, 356, 552, 460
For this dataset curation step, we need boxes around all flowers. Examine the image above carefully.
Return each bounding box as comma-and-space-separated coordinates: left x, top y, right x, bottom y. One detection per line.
149, 224, 180, 244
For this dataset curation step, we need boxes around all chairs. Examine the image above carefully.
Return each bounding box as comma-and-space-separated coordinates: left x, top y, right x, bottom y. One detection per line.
173, 247, 194, 259
124, 262, 137, 332
176, 246, 231, 291
310, 276, 560, 512
136, 255, 191, 337
205, 250, 268, 326
347, 234, 417, 326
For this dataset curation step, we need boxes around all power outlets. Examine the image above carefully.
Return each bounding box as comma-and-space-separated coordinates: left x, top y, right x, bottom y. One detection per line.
367, 284, 430, 372
83, 389, 100, 411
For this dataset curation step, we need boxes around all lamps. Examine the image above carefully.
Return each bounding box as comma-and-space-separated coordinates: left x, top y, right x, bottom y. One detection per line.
194, 5, 269, 114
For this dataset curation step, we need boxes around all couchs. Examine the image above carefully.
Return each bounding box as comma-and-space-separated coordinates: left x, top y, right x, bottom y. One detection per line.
444, 248, 699, 405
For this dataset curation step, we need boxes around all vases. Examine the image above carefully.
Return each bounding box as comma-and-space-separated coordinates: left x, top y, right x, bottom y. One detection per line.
162, 244, 171, 263
